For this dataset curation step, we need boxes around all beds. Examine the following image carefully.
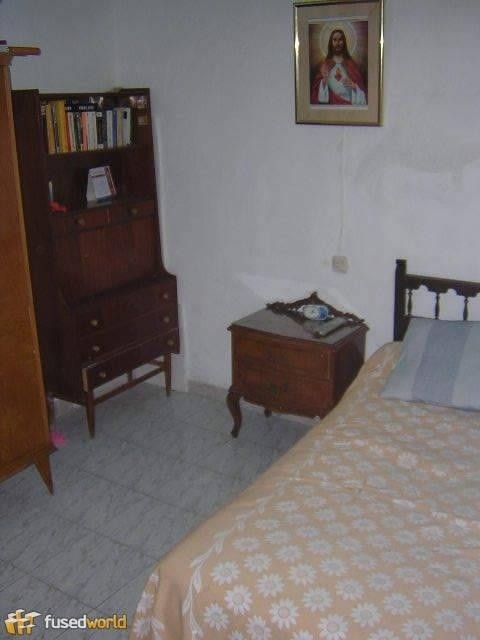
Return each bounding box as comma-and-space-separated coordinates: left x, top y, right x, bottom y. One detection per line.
154, 257, 480, 640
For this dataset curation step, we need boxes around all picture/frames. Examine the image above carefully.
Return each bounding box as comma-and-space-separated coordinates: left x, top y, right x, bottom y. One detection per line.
292, 0, 386, 128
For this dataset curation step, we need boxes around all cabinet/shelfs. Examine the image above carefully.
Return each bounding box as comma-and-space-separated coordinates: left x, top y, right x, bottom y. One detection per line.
11, 87, 181, 440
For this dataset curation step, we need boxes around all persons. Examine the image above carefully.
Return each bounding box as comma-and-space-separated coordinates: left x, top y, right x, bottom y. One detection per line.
311, 29, 368, 105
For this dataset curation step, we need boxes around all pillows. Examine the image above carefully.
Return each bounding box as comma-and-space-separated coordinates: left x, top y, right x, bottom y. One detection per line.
378, 316, 480, 412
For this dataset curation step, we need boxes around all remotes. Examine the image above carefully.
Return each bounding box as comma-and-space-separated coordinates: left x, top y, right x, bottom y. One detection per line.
315, 318, 347, 336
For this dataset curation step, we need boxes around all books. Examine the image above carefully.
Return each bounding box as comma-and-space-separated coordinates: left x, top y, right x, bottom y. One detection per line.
39, 96, 135, 156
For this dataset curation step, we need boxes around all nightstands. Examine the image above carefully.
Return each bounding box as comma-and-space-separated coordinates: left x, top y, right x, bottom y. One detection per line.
225, 302, 370, 440
